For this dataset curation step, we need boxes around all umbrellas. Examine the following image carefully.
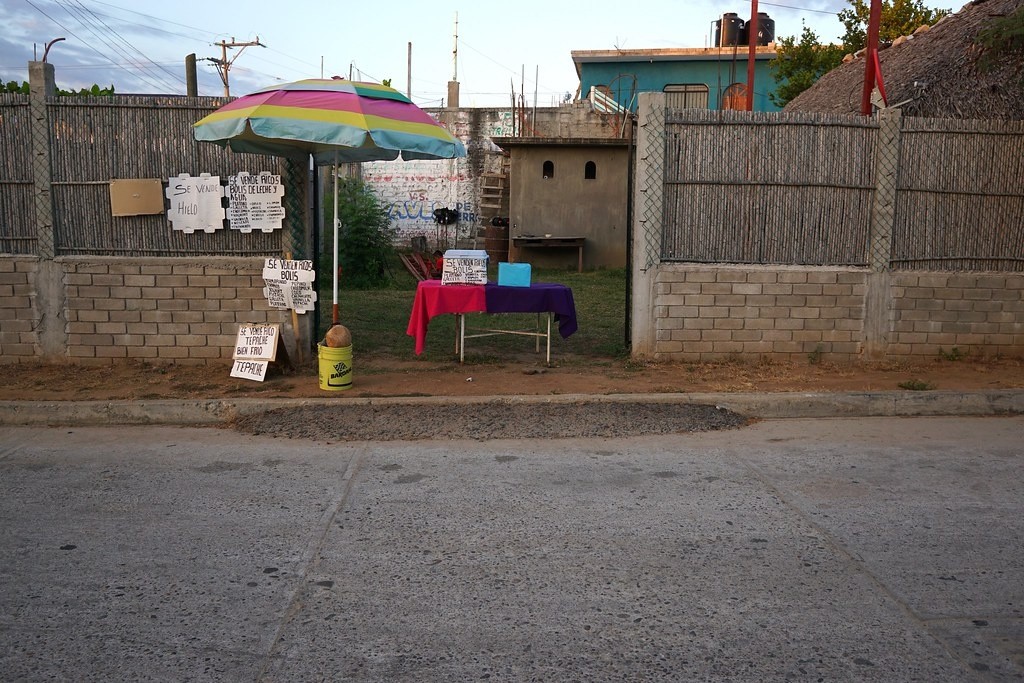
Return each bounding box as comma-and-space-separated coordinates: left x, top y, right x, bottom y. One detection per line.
189, 75, 470, 327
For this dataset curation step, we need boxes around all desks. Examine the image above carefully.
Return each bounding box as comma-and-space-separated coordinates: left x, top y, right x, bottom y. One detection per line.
406, 279, 578, 363
511, 236, 587, 274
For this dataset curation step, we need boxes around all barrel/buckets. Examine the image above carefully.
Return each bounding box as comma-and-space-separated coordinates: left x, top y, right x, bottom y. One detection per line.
318, 343, 354, 391
